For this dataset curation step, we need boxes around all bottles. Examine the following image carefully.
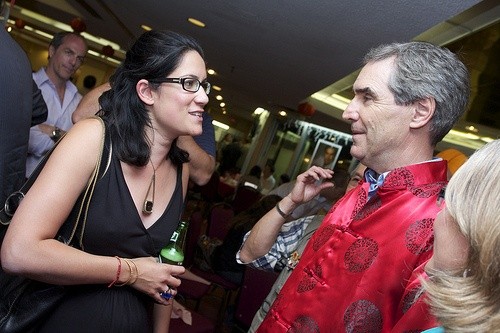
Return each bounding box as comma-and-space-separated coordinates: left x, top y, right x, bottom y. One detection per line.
155, 219, 189, 306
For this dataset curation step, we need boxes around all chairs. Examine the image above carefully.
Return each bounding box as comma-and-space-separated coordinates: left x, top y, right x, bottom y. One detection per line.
170, 172, 262, 333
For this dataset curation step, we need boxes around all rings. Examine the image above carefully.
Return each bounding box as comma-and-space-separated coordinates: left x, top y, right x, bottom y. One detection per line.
165, 286, 174, 299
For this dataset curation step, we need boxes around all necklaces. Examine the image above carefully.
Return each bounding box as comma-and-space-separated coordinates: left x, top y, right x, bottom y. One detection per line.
141, 150, 166, 216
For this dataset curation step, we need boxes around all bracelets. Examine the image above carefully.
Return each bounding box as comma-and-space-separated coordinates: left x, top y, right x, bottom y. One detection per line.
108, 256, 139, 289
275, 200, 295, 218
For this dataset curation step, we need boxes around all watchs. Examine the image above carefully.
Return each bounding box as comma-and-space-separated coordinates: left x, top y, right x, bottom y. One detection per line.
50, 125, 63, 142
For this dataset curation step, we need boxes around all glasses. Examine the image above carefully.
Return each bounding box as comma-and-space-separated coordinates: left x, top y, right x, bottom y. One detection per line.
148, 78, 211, 95
347, 174, 360, 188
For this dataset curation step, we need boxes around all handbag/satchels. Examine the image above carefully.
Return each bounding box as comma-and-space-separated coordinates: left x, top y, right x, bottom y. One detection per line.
191, 235, 223, 265
0, 115, 110, 333
197, 283, 241, 323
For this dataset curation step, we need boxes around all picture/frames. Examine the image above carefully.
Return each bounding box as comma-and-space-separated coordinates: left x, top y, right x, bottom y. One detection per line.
308, 139, 343, 171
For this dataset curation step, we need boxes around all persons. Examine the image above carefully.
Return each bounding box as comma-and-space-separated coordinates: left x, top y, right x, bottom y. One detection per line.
0, 23, 472, 292
0, 29, 209, 333
400, 139, 500, 333
253, 40, 470, 333
